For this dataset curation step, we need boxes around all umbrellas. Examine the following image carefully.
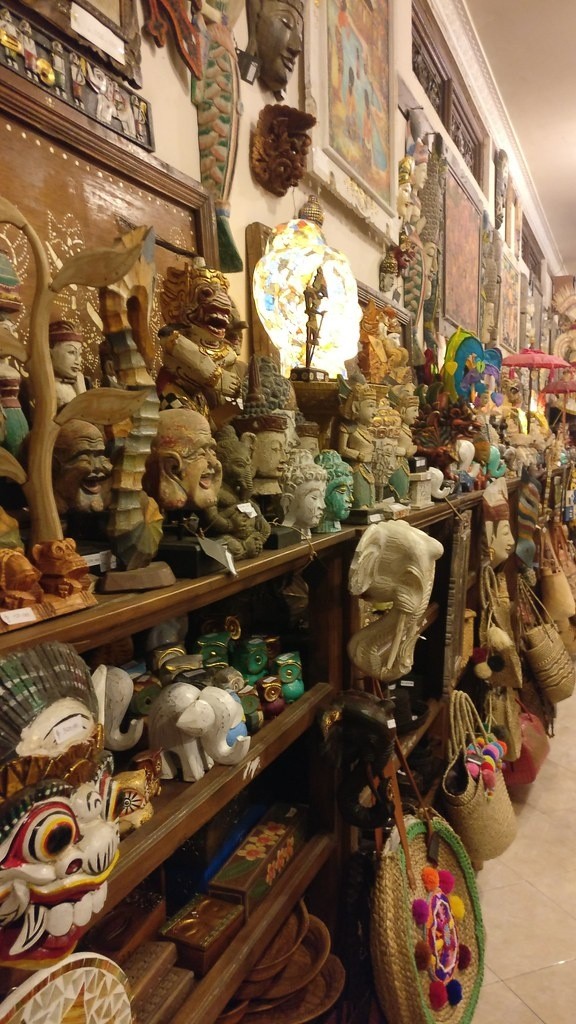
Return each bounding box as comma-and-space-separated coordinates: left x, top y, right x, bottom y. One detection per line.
501, 336, 576, 434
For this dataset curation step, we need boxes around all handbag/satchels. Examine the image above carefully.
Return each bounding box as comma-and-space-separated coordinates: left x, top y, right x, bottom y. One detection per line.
497, 697, 550, 787
517, 578, 574, 704
485, 688, 521, 762
540, 516, 576, 620
370, 808, 485, 1024
478, 565, 523, 688
443, 690, 519, 862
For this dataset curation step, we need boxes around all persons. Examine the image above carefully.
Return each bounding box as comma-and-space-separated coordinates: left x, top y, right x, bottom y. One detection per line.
47, 319, 419, 534
246, 0, 307, 91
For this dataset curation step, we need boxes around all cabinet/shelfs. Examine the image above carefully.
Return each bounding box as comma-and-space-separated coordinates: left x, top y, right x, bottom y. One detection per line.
0, 461, 576, 1024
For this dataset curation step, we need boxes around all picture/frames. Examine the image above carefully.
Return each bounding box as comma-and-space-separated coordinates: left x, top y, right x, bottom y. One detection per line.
245, 221, 414, 374
442, 159, 483, 340
525, 282, 543, 350
499, 252, 521, 354
0, 60, 220, 388
304, 0, 400, 247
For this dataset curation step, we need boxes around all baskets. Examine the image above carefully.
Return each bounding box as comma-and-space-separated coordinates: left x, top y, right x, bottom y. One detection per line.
217, 899, 346, 1024
